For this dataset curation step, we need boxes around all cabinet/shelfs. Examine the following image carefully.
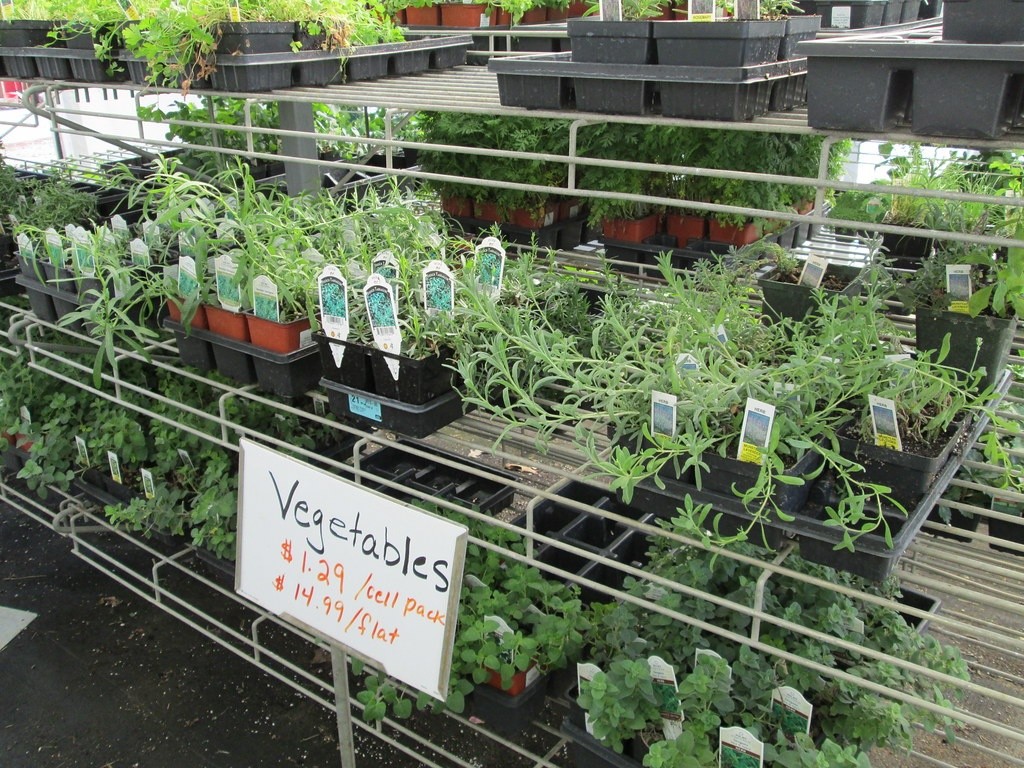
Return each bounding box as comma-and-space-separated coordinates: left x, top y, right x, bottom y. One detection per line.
4, 21, 1022, 764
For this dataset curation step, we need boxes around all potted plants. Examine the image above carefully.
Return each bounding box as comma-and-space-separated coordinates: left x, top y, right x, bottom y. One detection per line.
2, 2, 1022, 767
